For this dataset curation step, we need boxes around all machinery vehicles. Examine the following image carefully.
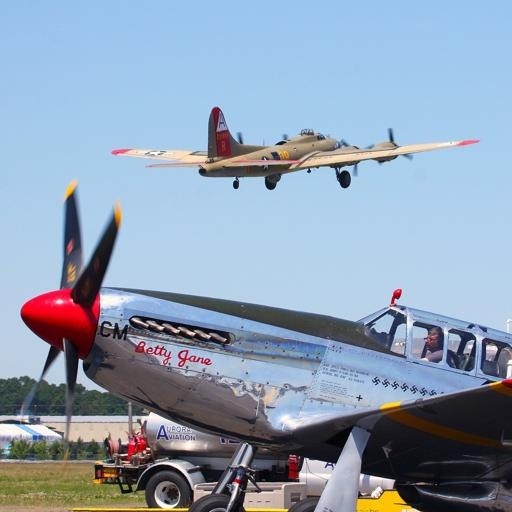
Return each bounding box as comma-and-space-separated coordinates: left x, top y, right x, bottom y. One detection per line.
85, 408, 395, 510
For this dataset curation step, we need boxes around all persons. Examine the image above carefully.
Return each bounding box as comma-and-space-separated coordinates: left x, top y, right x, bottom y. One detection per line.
423, 327, 443, 363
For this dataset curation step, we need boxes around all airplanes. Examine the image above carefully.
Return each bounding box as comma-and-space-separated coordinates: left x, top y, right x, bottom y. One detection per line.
13, 174, 511, 512
108, 107, 481, 196
